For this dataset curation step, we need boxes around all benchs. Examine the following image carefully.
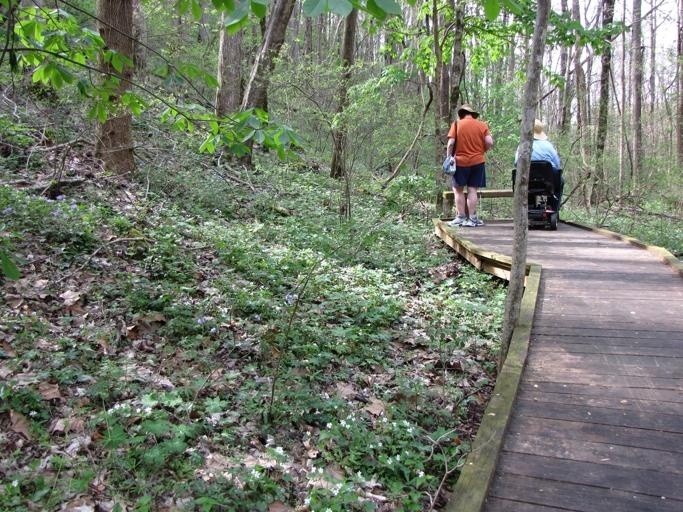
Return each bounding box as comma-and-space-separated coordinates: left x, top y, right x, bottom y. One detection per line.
440, 189, 515, 221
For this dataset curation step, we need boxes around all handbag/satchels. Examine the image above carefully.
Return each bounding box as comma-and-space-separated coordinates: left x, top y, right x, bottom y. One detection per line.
442, 155, 457, 176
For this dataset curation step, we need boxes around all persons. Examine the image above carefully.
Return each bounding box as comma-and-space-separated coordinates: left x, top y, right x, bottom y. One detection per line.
513, 118, 565, 223
446, 104, 494, 228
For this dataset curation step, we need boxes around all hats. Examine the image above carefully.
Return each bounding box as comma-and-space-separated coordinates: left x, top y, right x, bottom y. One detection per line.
458, 104, 479, 120
534, 119, 546, 139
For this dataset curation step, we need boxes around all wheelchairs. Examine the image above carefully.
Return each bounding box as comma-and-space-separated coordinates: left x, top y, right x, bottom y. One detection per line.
508, 161, 566, 230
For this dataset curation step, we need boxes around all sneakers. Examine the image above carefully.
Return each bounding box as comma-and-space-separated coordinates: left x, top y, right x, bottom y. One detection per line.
448, 214, 467, 226
468, 217, 484, 227
